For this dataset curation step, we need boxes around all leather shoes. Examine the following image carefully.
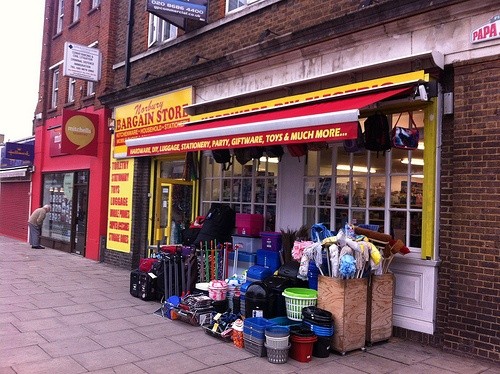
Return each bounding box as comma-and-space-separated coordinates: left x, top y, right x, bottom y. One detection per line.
32, 245, 45, 249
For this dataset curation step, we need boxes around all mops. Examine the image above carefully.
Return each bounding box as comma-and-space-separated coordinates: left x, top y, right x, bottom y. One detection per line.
290, 227, 383, 281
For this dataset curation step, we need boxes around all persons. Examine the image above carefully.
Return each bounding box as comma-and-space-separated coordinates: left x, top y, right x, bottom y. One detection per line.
26, 205, 51, 250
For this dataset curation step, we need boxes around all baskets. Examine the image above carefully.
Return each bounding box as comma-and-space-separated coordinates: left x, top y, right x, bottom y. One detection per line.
242, 317, 303, 364
282, 292, 317, 320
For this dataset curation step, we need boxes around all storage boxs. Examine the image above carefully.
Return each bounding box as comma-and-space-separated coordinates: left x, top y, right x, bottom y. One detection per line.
365, 271, 395, 345
318, 274, 367, 356
228, 232, 334, 364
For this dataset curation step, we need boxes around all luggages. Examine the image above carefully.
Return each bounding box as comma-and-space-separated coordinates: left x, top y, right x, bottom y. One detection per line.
130, 249, 178, 304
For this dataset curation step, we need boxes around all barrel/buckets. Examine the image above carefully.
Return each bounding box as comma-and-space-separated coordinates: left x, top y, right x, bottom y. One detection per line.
289, 306, 335, 363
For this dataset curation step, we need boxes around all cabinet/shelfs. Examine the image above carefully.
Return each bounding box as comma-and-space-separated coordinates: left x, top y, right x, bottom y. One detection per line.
47, 186, 72, 237
223, 165, 422, 248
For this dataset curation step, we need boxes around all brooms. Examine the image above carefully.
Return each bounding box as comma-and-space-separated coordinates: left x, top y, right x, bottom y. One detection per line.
350, 221, 413, 276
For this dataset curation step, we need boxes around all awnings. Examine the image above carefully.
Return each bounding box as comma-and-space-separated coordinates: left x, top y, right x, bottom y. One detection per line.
125, 88, 412, 159
